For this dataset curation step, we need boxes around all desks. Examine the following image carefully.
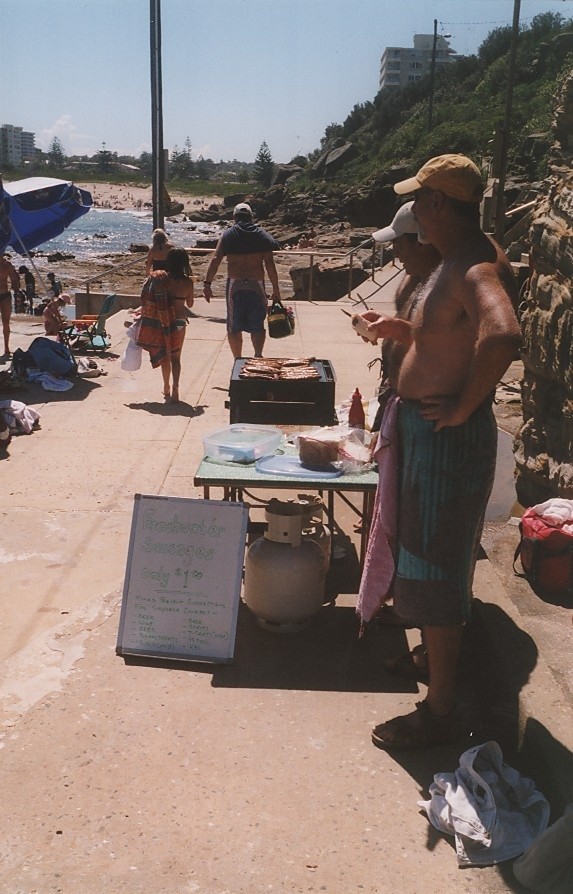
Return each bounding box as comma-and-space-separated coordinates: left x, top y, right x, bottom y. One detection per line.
194, 445, 380, 607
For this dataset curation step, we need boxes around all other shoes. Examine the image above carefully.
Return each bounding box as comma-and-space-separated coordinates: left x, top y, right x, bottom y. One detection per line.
374, 606, 423, 627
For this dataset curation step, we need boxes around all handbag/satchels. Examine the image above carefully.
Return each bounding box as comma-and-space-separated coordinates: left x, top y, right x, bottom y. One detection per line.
417, 740, 551, 866
512, 498, 573, 593
268, 300, 296, 339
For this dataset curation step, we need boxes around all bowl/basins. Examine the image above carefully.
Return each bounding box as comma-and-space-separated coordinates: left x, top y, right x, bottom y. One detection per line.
201, 422, 282, 464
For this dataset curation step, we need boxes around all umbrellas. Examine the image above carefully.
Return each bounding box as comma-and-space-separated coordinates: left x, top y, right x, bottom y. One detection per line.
0, 175, 95, 291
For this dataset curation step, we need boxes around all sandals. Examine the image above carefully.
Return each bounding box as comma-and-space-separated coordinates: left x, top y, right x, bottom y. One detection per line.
393, 642, 470, 676
370, 699, 461, 749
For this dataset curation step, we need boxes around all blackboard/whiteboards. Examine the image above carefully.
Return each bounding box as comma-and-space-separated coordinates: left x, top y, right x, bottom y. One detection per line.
118, 492, 248, 666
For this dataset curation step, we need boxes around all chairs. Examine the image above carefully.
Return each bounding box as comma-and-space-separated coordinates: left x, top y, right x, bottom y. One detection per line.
58, 294, 117, 355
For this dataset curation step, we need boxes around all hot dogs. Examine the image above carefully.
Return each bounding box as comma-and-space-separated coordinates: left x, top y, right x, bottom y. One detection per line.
351, 313, 378, 344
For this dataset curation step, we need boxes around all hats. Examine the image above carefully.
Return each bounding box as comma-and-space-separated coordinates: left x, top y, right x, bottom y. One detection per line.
372, 201, 419, 242
59, 294, 71, 305
394, 153, 484, 203
233, 203, 252, 217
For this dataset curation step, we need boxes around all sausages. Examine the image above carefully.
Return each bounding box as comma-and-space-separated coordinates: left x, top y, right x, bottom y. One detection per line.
239, 356, 322, 381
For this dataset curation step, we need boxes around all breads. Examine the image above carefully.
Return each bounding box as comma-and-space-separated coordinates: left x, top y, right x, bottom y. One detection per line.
297, 435, 371, 467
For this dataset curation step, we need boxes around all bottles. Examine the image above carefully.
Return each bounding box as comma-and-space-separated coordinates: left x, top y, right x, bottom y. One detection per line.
348, 387, 365, 445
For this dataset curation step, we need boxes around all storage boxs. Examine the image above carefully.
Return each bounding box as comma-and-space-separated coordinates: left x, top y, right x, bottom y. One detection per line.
59, 305, 76, 319
204, 423, 283, 463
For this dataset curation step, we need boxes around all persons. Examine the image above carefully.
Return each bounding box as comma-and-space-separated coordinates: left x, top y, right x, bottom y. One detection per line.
362, 199, 443, 679
93, 200, 96, 208
19, 265, 35, 309
43, 294, 72, 335
47, 280, 59, 297
137, 200, 141, 210
203, 202, 281, 359
357, 152, 523, 750
0, 255, 21, 353
144, 228, 172, 278
135, 247, 193, 403
